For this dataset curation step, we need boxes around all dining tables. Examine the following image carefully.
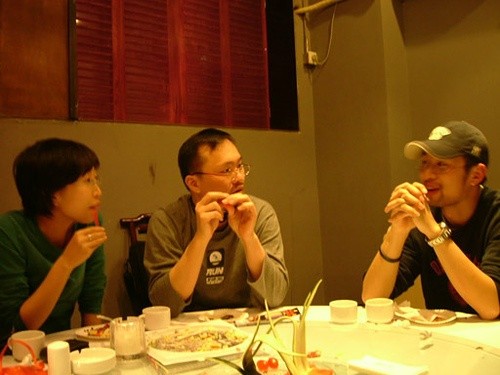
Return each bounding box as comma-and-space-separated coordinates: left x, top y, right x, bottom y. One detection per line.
0, 305, 500, 375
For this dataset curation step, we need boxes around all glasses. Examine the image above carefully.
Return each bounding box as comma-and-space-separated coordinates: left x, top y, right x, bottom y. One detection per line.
190, 162, 250, 181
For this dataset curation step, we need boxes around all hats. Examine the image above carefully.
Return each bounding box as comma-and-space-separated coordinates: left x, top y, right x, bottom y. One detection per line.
404, 122, 488, 167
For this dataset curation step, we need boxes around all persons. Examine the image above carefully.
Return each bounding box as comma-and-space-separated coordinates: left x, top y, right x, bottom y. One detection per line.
362, 122, 500, 321
144, 128, 289, 319
0, 138, 107, 335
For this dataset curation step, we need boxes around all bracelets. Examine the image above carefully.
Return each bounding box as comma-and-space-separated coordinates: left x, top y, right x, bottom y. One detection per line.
378, 245, 402, 263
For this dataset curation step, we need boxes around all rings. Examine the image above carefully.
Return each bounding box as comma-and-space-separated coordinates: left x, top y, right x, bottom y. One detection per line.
89, 234, 93, 241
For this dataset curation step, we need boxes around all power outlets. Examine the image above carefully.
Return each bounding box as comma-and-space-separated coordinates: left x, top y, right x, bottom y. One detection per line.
303, 51, 318, 66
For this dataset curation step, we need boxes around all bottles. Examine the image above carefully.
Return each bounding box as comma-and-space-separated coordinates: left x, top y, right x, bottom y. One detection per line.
47, 341, 71, 375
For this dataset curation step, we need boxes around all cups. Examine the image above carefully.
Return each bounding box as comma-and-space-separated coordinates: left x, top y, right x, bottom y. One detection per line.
10, 330, 46, 360
365, 298, 393, 323
329, 298, 357, 324
110, 316, 146, 359
143, 305, 171, 330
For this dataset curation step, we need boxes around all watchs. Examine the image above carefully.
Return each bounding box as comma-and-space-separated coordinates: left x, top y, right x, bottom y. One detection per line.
425, 222, 451, 246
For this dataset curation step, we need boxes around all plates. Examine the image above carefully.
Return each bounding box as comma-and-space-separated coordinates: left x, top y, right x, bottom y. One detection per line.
142, 318, 255, 367
408, 309, 457, 324
74, 323, 110, 340
172, 308, 249, 325
